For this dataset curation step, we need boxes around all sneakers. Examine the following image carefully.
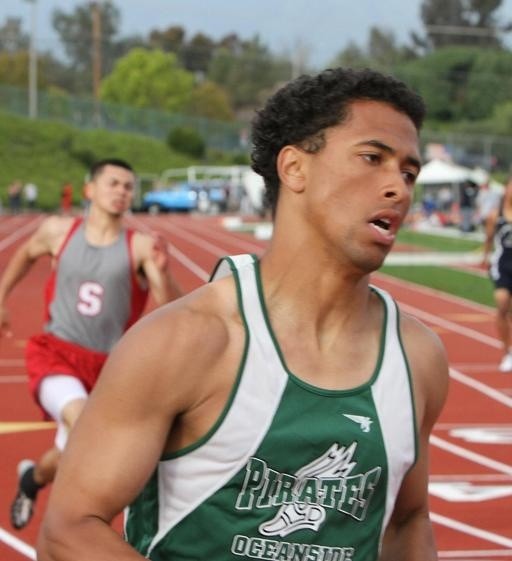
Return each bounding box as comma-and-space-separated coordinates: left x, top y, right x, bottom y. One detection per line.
11, 456, 44, 528
499, 353, 512, 375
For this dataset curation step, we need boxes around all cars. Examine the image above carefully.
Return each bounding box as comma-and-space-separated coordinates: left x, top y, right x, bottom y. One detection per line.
142, 182, 232, 216
425, 135, 512, 173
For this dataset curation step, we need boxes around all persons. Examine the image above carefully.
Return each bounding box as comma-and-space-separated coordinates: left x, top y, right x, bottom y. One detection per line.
8, 180, 20, 214
38, 70, 449, 561
421, 179, 512, 373
61, 183, 73, 214
24, 183, 36, 213
0, 160, 176, 529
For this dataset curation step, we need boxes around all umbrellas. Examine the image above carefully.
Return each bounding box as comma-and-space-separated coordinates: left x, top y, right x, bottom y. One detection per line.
415, 159, 471, 184
